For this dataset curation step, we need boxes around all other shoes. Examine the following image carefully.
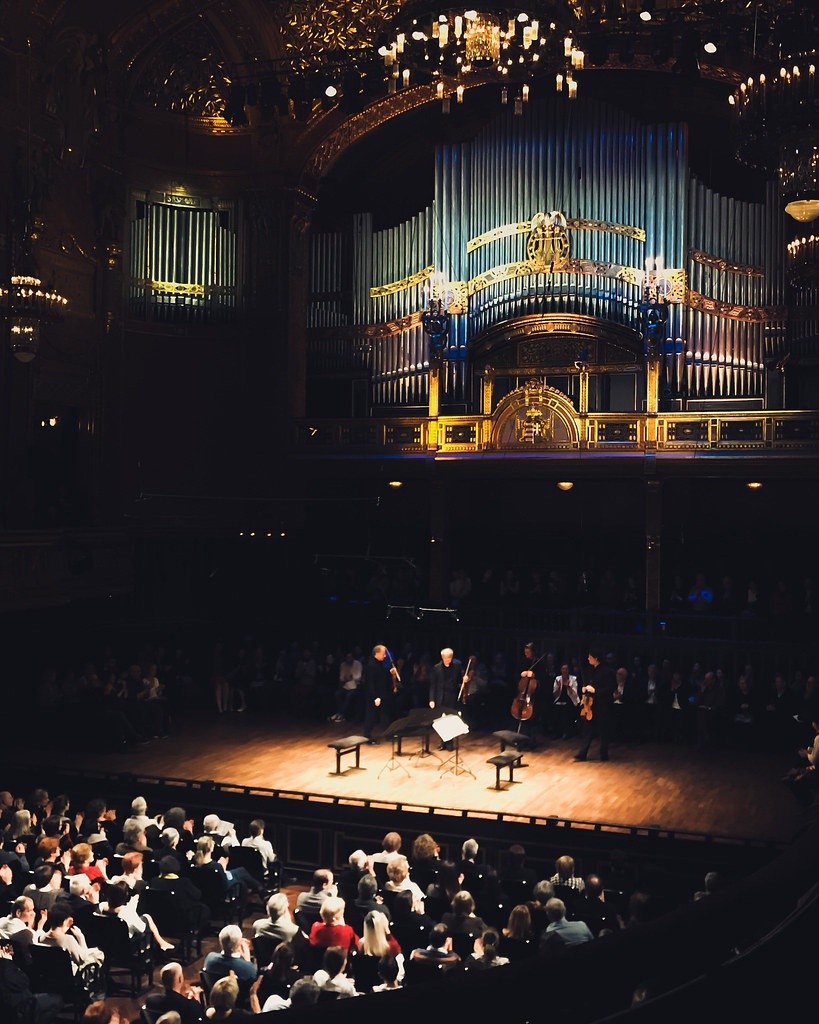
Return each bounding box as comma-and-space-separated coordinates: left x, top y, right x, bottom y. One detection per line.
365, 734, 375, 745
237, 706, 247, 712
448, 742, 453, 750
601, 753, 609, 761
440, 741, 446, 749
573, 754, 586, 760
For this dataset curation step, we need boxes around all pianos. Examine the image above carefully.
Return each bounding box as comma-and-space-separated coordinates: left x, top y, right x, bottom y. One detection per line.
383, 704, 476, 783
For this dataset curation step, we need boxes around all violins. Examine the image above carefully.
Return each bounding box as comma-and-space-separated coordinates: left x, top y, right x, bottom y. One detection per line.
579, 689, 596, 723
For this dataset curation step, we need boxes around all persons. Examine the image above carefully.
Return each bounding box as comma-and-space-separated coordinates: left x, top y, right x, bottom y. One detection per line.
0, 787, 752, 1024
23, 560, 818, 780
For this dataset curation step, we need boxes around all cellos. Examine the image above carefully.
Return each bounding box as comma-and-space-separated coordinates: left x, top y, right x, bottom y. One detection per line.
511, 657, 540, 734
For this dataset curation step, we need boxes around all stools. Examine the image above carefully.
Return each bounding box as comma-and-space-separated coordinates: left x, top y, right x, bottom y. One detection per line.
326, 734, 370, 776
486, 730, 529, 790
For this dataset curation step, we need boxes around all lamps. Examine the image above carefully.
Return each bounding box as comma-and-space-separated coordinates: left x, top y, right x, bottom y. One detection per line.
763, 353, 785, 373
220, 44, 391, 127
717, 6, 819, 258
374, 6, 586, 116
359, 153, 438, 235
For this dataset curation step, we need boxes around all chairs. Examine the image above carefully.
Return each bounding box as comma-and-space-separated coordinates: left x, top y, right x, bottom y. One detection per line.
0, 806, 626, 1024
